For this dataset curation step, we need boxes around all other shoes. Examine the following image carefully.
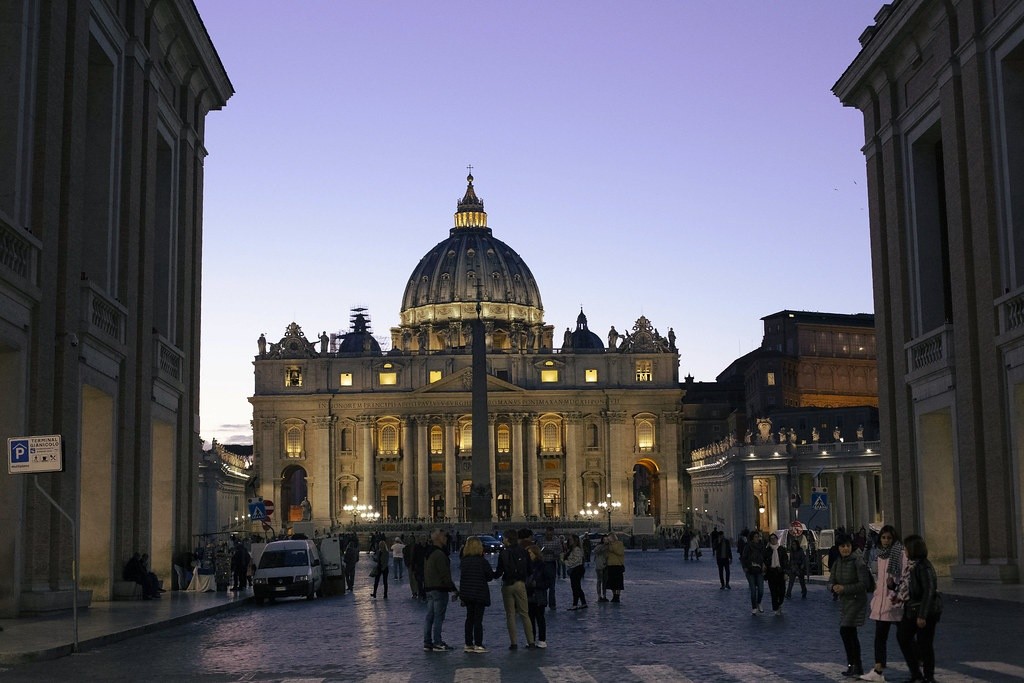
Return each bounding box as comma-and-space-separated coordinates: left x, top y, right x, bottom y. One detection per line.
537, 641, 547, 648
526, 644, 535, 649
860, 669, 884, 683
510, 644, 518, 650
841, 664, 863, 677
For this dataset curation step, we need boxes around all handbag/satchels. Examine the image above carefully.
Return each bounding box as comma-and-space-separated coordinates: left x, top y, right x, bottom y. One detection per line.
621, 565, 626, 572
866, 571, 874, 593
698, 551, 703, 557
369, 568, 378, 577
906, 602, 920, 617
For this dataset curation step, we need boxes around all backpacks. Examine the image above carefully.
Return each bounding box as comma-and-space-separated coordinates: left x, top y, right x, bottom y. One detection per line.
507, 548, 533, 582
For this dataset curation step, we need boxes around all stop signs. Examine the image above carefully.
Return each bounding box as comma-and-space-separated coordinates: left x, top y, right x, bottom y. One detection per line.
262, 516, 272, 531
262, 499, 275, 515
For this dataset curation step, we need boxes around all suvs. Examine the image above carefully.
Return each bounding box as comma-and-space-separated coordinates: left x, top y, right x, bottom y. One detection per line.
475, 534, 502, 553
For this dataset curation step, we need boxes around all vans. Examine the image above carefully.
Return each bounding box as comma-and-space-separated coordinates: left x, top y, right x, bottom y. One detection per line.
768, 529, 818, 570
252, 539, 323, 606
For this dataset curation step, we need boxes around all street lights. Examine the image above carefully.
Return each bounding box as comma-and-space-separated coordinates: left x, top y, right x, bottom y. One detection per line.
342, 493, 364, 526
598, 492, 622, 532
579, 501, 599, 533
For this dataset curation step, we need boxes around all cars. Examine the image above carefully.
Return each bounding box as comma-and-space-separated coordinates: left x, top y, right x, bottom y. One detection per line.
580, 533, 605, 549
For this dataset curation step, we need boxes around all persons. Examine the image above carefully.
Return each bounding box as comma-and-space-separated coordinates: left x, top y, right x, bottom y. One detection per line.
636, 492, 648, 514
123, 553, 166, 601
833, 426, 841, 440
593, 532, 625, 603
737, 525, 827, 617
608, 326, 618, 347
299, 497, 311, 521
669, 327, 676, 346
744, 429, 753, 444
258, 334, 266, 354
173, 532, 291, 593
887, 534, 937, 683
828, 526, 881, 601
856, 424, 864, 439
318, 331, 329, 352
691, 432, 736, 461
564, 328, 571, 346
859, 525, 910, 683
710, 527, 718, 556
778, 427, 820, 442
714, 531, 733, 590
339, 530, 588, 654
828, 534, 869, 677
660, 526, 710, 561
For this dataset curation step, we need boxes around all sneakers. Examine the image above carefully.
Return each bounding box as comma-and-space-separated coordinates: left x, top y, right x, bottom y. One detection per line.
473, 646, 491, 653
464, 644, 474, 653
424, 644, 431, 651
433, 641, 454, 652
